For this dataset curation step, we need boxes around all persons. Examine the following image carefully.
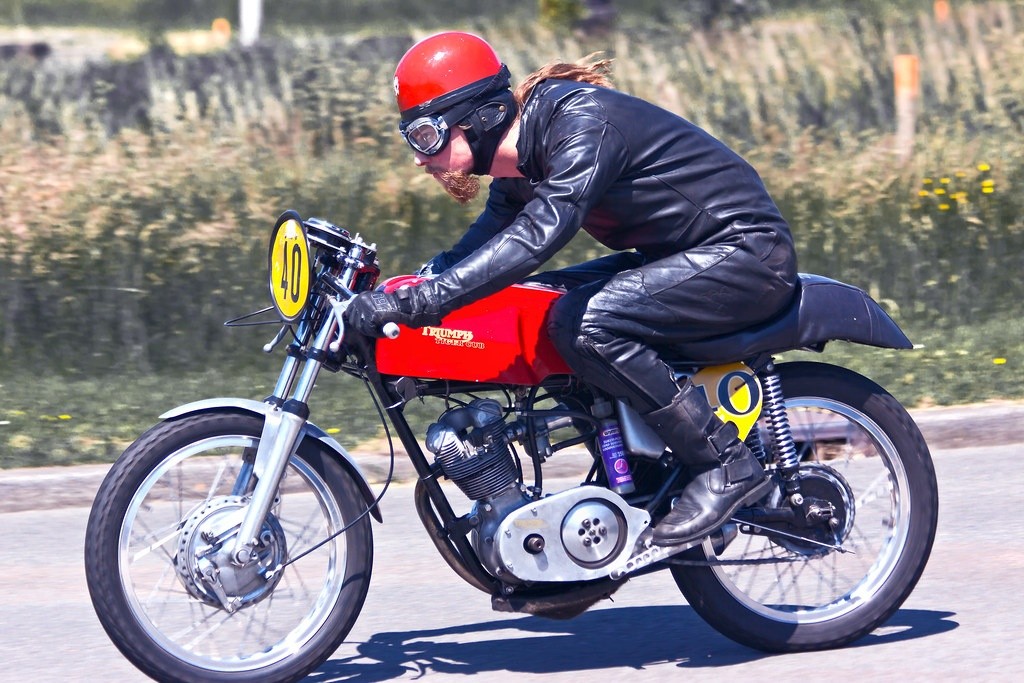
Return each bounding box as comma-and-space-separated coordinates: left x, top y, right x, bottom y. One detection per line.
344, 31, 798, 548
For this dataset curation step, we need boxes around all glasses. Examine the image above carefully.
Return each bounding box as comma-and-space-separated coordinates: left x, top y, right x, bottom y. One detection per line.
396, 117, 451, 156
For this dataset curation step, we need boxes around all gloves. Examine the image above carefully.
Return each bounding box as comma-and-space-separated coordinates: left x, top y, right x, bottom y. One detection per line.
339, 282, 442, 339
412, 251, 448, 276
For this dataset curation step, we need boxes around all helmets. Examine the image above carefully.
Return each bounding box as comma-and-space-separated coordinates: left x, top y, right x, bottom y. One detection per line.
393, 31, 510, 120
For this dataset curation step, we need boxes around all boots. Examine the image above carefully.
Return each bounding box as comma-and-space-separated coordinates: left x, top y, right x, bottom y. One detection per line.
639, 375, 773, 546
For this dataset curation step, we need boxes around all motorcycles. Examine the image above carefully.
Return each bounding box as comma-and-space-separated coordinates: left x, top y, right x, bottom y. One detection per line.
81, 208, 940, 682
